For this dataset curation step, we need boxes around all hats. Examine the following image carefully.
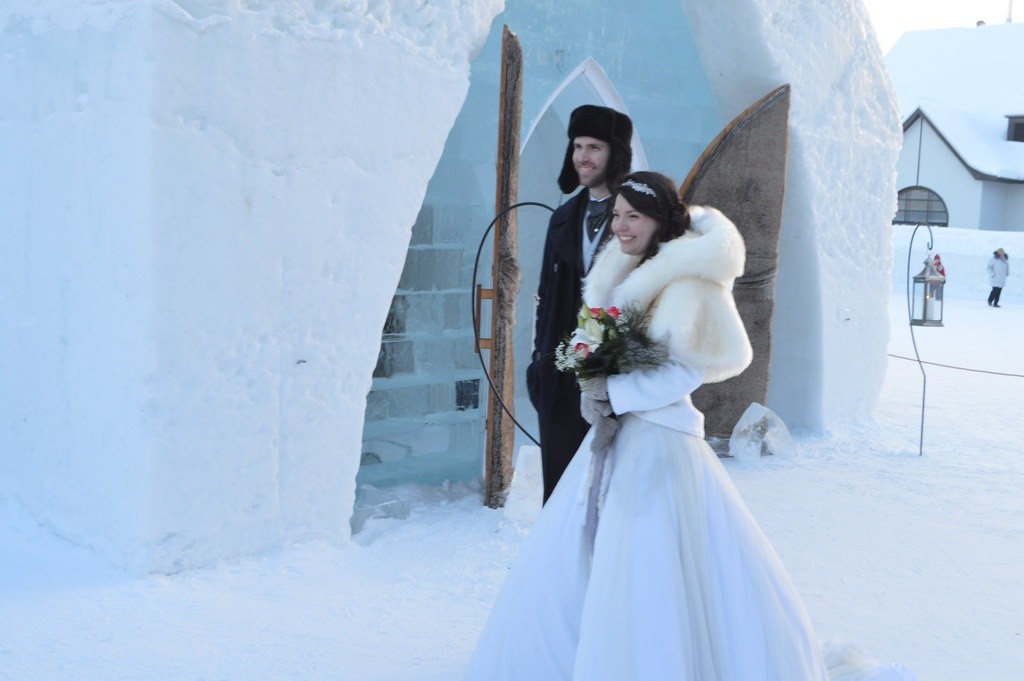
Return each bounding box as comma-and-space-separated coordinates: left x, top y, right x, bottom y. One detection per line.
997, 248, 1004, 253
558, 104, 632, 195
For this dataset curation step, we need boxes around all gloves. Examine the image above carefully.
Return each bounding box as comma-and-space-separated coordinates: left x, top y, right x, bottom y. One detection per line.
580, 392, 613, 425
578, 374, 609, 401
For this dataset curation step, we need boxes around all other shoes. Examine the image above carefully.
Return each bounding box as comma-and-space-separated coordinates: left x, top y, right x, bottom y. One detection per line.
994, 305, 1000, 308
988, 301, 991, 305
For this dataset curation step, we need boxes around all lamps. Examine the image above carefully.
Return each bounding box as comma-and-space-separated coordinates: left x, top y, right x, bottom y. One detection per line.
912, 256, 946, 326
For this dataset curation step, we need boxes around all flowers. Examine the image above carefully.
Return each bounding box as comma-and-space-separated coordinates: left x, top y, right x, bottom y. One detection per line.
552, 299, 675, 378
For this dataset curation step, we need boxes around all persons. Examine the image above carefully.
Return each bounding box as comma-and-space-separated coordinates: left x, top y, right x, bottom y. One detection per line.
526, 105, 633, 508
457, 172, 827, 680
987, 248, 1009, 307
927, 254, 945, 300
